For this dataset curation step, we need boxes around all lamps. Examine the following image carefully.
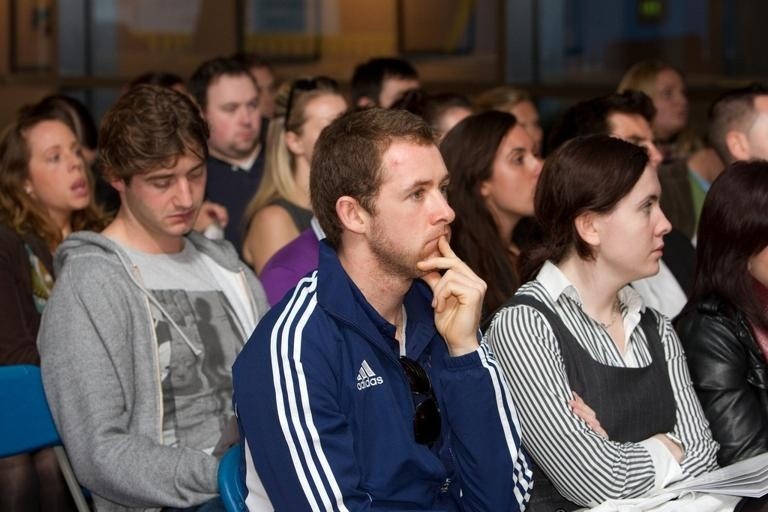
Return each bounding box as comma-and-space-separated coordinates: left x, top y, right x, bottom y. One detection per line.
0, 363, 249, 512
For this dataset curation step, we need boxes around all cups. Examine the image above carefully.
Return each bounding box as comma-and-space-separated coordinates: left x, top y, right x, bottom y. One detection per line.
394, 352, 443, 444
285, 75, 341, 132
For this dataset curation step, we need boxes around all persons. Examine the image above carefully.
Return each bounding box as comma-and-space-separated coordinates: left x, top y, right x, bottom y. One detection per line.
539, 61, 768, 318
232, 106, 533, 508
33, 85, 269, 509
256, 56, 547, 332
23, 96, 120, 226
236, 75, 348, 275
186, 59, 274, 253
125, 72, 206, 123
675, 161, 768, 510
481, 134, 719, 508
2, 112, 115, 508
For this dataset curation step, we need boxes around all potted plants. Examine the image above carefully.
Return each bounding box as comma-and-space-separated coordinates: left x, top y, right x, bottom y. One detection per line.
397, 1, 475, 58
8, 1, 57, 72
234, 1, 323, 64
